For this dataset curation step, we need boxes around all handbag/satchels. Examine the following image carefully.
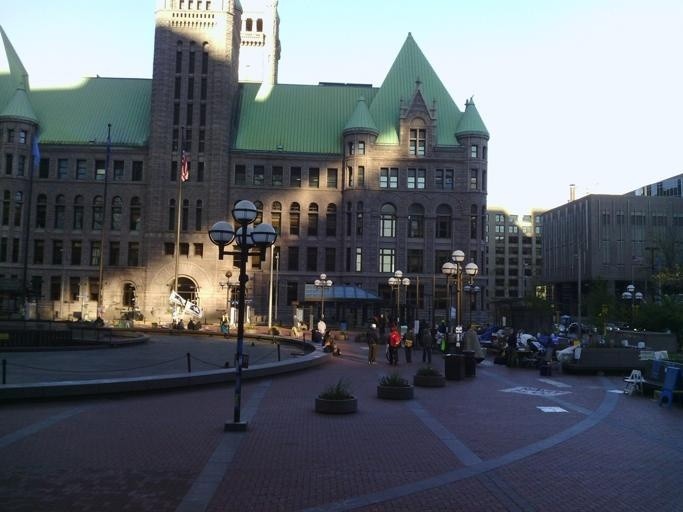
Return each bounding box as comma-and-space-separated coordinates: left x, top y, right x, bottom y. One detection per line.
405, 339, 413, 347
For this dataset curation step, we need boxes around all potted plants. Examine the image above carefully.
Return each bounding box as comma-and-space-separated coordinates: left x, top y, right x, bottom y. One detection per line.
315, 376, 358, 414
377, 370, 413, 399
414, 365, 445, 387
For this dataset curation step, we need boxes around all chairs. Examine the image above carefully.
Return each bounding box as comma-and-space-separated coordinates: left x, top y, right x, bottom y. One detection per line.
623, 369, 646, 396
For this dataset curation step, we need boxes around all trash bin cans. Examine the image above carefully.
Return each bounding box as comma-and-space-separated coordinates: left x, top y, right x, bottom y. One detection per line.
442, 350, 475, 379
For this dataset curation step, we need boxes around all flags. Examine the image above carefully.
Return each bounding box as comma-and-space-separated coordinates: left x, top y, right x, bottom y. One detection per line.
181, 132, 189, 183
31, 125, 41, 179
105, 135, 113, 179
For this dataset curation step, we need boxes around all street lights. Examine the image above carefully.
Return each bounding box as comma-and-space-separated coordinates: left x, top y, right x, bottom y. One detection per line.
219, 270, 238, 316
621, 284, 642, 330
387, 269, 411, 331
208, 199, 279, 423
314, 273, 333, 320
442, 249, 479, 352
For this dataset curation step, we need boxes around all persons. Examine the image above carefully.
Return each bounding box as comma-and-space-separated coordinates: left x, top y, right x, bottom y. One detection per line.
220, 311, 230, 334
317, 310, 557, 370
579, 334, 593, 347
177, 318, 202, 330
125, 318, 130, 328
